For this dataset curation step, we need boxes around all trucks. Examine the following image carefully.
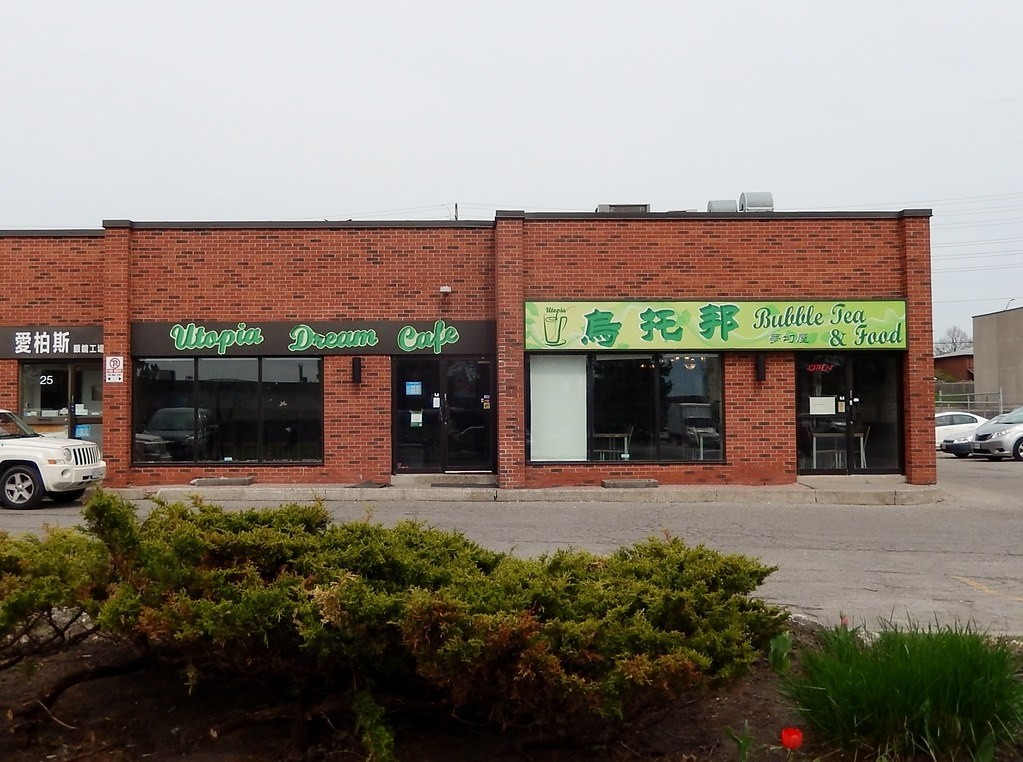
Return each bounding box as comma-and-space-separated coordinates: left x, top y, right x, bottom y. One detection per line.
667, 402, 720, 449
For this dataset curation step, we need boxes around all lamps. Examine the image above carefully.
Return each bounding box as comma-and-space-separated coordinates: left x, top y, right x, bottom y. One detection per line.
683, 357, 696, 369
439, 285, 453, 293
352, 355, 361, 383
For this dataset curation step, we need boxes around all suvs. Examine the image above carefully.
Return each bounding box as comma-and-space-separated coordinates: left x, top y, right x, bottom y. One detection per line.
0, 409, 107, 509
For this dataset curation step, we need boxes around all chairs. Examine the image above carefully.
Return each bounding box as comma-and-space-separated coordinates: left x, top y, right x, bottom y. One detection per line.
806, 425, 872, 467
591, 425, 635, 460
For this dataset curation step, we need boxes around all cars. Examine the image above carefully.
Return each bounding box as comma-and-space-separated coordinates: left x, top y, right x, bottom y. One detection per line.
143, 407, 218, 459
935, 412, 989, 450
659, 427, 671, 440
970, 407, 1023, 460
452, 426, 488, 446
940, 414, 1009, 460
135, 433, 172, 461
797, 414, 856, 457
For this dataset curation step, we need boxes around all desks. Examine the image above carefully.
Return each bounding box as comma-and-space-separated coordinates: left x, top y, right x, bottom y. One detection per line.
593, 432, 633, 459
813, 431, 867, 470
